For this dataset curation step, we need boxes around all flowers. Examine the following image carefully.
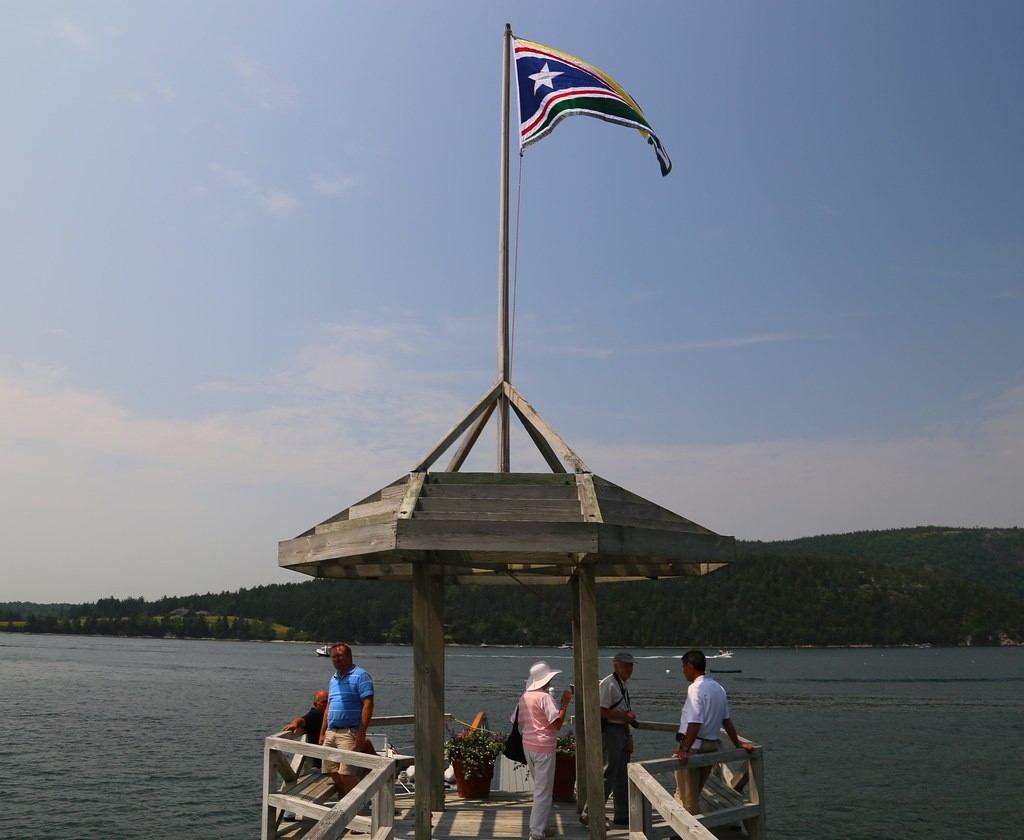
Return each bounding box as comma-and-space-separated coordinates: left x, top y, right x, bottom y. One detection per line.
444, 728, 508, 781
556, 729, 579, 758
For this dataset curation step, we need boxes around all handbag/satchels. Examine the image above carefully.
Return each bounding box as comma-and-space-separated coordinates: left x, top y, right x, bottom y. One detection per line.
504, 729, 530, 765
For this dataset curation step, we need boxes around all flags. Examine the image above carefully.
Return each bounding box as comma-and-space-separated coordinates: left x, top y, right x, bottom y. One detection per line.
512, 34, 672, 178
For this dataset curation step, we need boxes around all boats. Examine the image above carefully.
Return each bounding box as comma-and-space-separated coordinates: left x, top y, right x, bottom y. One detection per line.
316, 646, 330, 657
715, 650, 734, 658
480, 643, 489, 648
559, 643, 573, 649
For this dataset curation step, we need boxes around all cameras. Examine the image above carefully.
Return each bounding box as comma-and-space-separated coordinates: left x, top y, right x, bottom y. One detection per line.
630, 719, 641, 730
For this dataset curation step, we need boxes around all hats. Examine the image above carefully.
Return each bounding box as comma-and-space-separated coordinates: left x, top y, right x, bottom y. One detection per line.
526, 661, 563, 692
613, 653, 638, 665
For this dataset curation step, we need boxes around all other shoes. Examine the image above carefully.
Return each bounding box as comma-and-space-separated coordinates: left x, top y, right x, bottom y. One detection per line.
529, 829, 556, 840
580, 816, 609, 829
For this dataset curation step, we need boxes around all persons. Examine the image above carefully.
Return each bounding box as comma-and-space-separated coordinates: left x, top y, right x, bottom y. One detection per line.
281, 689, 377, 801
318, 644, 375, 835
579, 652, 636, 830
671, 650, 753, 816
510, 660, 573, 840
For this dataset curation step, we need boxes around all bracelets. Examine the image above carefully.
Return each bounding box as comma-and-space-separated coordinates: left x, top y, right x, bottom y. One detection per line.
560, 705, 567, 711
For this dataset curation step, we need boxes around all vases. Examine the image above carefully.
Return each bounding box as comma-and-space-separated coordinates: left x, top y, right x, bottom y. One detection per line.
451, 753, 494, 798
551, 752, 576, 802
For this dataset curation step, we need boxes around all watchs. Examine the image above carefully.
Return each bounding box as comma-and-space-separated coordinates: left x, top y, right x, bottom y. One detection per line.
679, 744, 690, 752
